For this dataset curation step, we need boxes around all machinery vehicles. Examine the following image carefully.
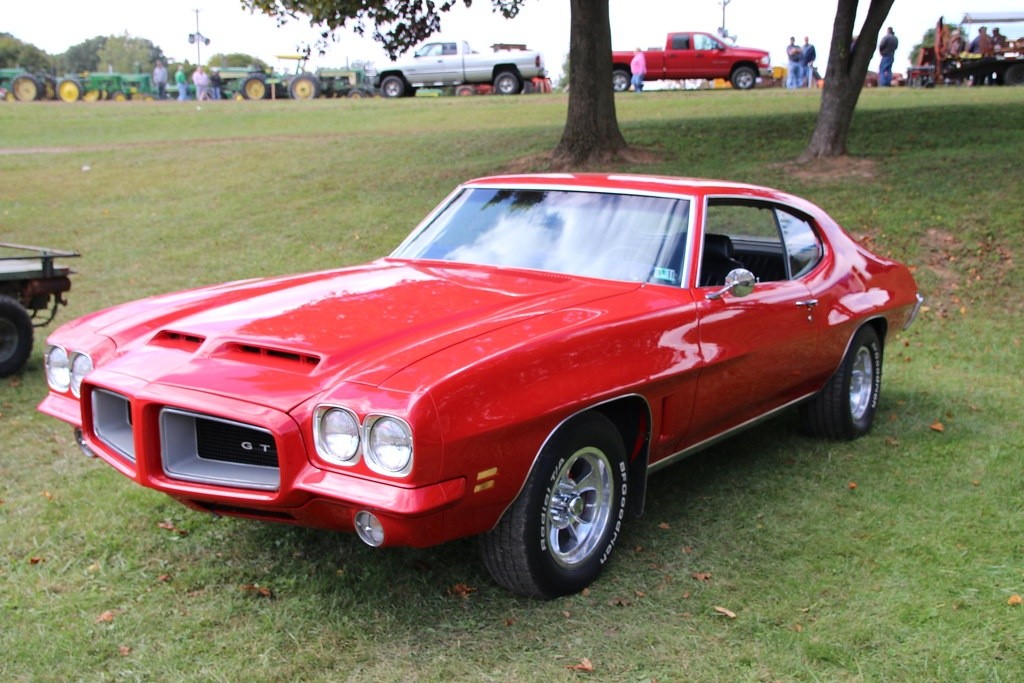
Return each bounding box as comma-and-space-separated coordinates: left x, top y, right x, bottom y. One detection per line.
0, 54, 373, 102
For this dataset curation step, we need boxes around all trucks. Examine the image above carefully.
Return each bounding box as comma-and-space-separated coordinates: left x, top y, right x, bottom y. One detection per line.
960, 9, 1024, 85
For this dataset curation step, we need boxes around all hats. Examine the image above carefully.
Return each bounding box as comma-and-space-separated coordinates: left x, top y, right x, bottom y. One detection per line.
992, 27, 999, 30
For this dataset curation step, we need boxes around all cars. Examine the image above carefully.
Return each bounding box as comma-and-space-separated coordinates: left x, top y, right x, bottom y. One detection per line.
36, 171, 924, 603
864, 71, 907, 88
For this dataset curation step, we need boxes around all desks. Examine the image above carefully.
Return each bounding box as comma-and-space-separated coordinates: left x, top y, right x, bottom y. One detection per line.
907, 66, 935, 86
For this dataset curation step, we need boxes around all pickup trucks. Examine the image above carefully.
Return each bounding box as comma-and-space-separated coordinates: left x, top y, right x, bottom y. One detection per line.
613, 32, 772, 94
372, 41, 544, 98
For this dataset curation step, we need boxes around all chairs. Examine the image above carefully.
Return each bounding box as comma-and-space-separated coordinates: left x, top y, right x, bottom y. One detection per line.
663, 233, 743, 288
905, 46, 936, 88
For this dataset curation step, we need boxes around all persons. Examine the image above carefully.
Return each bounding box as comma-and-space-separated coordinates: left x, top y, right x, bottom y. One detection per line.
801, 36, 816, 88
210, 69, 222, 100
968, 27, 1007, 86
945, 29, 961, 85
192, 65, 210, 101
631, 47, 647, 92
879, 27, 898, 86
175, 64, 187, 100
153, 60, 168, 100
787, 36, 804, 88
